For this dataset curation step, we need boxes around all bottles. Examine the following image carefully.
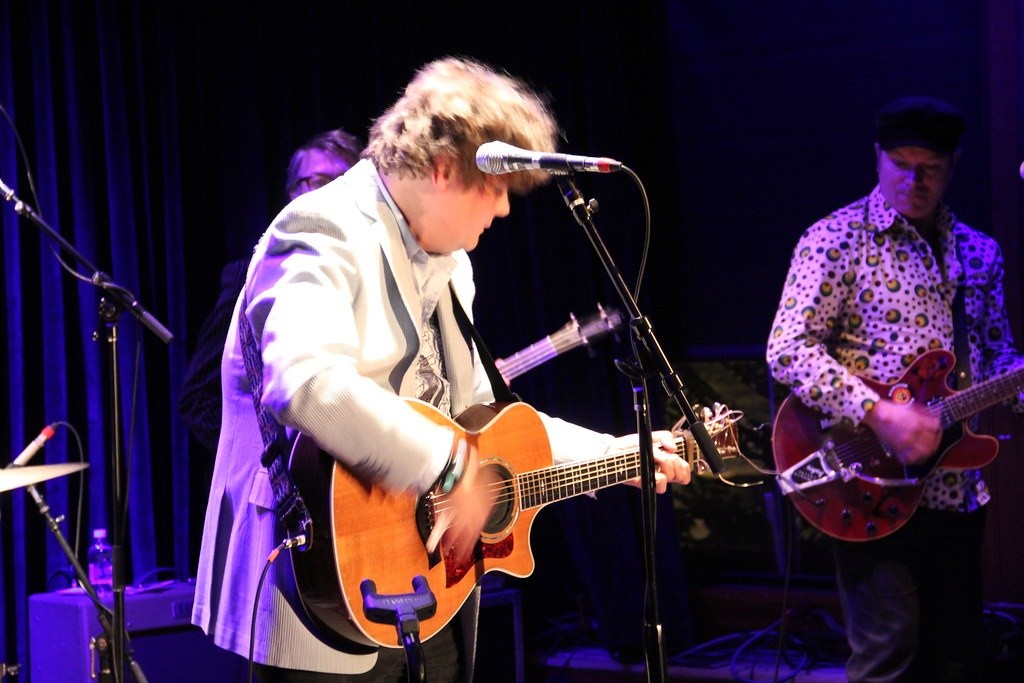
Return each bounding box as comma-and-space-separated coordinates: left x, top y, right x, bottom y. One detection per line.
87, 529, 114, 597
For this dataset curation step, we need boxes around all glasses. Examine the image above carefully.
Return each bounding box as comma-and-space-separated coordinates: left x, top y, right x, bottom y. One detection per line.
291, 174, 331, 190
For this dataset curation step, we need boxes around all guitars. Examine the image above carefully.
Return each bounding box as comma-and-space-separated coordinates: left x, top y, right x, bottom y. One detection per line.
269, 396, 738, 648
768, 349, 1022, 541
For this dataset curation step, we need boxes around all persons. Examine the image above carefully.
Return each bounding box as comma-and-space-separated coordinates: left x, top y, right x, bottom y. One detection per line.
190, 60, 691, 683
175, 129, 358, 450
766, 100, 1024, 683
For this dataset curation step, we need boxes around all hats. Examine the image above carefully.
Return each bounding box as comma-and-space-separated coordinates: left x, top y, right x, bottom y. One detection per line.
871, 98, 967, 154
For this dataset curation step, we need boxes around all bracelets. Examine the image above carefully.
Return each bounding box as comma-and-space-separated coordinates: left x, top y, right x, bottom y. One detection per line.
441, 439, 468, 493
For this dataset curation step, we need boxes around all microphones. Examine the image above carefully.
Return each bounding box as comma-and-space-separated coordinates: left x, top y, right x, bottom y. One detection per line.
475, 140, 624, 174
